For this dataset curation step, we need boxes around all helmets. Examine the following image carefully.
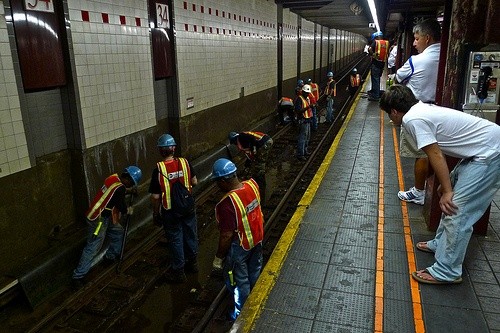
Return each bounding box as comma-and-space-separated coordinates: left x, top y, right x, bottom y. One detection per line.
228, 131, 240, 140
124, 166, 143, 187
371, 33, 377, 40
374, 31, 383, 38
302, 84, 312, 93
297, 79, 304, 86
158, 134, 177, 147
209, 157, 239, 179
352, 68, 358, 72
326, 71, 333, 77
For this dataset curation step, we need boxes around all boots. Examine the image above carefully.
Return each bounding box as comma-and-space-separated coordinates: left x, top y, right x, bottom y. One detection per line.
185, 256, 200, 274
175, 270, 186, 281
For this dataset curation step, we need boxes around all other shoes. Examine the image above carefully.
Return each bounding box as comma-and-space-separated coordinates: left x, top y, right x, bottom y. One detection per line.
298, 157, 307, 161
367, 97, 380, 102
305, 153, 312, 156
72, 280, 88, 290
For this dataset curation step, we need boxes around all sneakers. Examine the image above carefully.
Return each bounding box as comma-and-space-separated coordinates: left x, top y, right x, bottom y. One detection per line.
397, 186, 424, 206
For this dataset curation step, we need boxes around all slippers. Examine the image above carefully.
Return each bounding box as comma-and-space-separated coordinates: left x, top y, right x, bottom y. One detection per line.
411, 269, 463, 285
415, 241, 434, 254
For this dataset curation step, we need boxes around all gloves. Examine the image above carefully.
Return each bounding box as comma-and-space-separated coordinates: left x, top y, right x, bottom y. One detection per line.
212, 253, 226, 272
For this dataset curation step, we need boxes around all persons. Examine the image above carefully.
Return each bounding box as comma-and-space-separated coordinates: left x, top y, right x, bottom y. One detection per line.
212, 160, 265, 320
346, 68, 365, 103
296, 84, 316, 160
151, 133, 199, 282
367, 31, 390, 101
229, 131, 274, 178
379, 84, 500, 285
278, 96, 297, 125
307, 77, 320, 123
397, 18, 441, 204
387, 36, 397, 76
70, 166, 142, 287
298, 79, 318, 130
324, 72, 336, 123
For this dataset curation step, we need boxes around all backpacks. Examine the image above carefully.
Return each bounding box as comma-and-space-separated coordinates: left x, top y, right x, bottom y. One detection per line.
157, 159, 196, 225
